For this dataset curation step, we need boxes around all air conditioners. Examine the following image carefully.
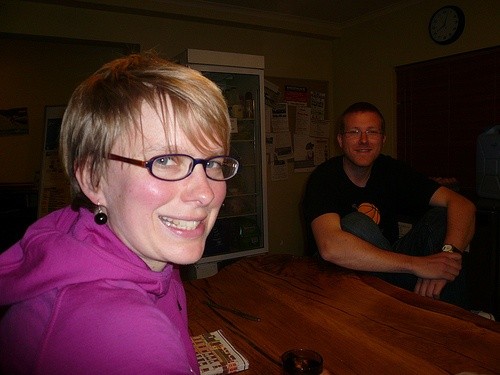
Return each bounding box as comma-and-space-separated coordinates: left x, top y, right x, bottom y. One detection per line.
36, 106, 76, 223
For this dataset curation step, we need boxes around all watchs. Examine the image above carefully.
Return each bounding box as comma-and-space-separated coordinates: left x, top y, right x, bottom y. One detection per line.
442, 244, 460, 253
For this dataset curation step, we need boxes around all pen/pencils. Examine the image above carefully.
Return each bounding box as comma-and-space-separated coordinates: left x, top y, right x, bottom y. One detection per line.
200, 300, 261, 322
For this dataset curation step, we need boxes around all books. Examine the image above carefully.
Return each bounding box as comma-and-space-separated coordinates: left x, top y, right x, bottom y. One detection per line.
190, 329, 249, 375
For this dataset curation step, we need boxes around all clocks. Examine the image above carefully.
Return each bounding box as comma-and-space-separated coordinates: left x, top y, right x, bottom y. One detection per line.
428, 5, 465, 45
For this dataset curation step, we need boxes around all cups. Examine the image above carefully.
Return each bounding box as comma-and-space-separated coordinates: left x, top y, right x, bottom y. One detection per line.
281, 348, 322, 375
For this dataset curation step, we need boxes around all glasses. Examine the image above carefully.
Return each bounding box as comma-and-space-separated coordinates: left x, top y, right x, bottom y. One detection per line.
92, 149, 243, 181
340, 128, 386, 137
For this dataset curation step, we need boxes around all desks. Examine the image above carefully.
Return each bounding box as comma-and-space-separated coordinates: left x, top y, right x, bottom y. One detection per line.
181, 252, 500, 375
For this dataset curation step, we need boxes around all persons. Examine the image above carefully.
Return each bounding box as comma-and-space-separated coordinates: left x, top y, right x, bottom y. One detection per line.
1, 55, 240, 375
305, 101, 476, 299
